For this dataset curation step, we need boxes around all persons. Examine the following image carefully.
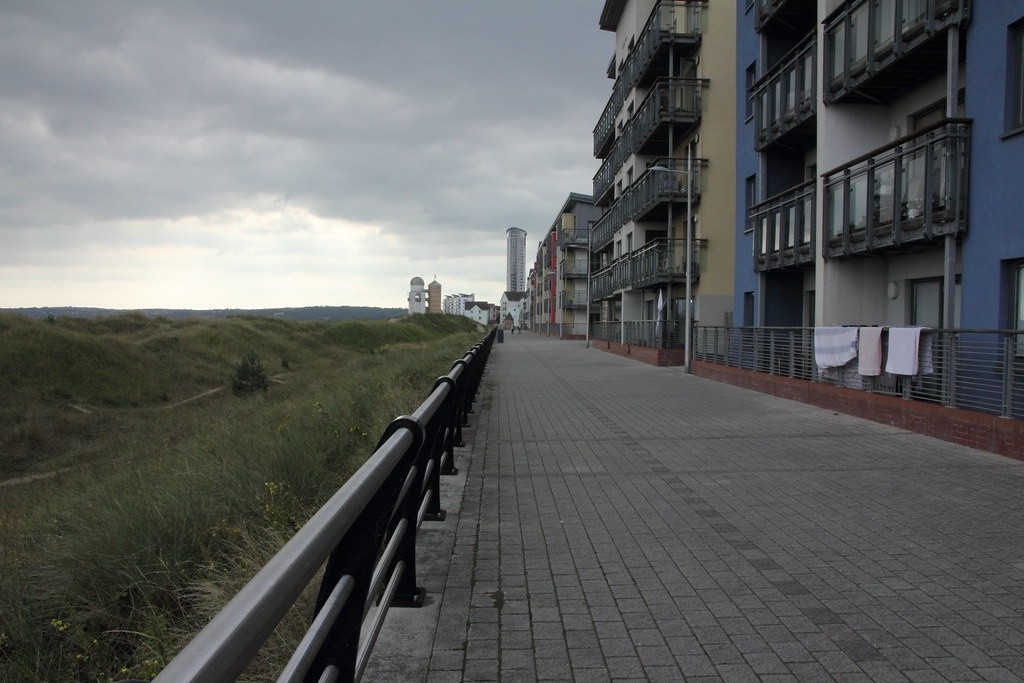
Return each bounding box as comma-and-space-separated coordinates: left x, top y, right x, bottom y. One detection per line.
511, 324, 514, 333
517, 324, 521, 335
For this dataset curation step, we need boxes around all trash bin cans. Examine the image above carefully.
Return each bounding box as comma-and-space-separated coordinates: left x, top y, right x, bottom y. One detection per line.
497, 324, 504, 343
